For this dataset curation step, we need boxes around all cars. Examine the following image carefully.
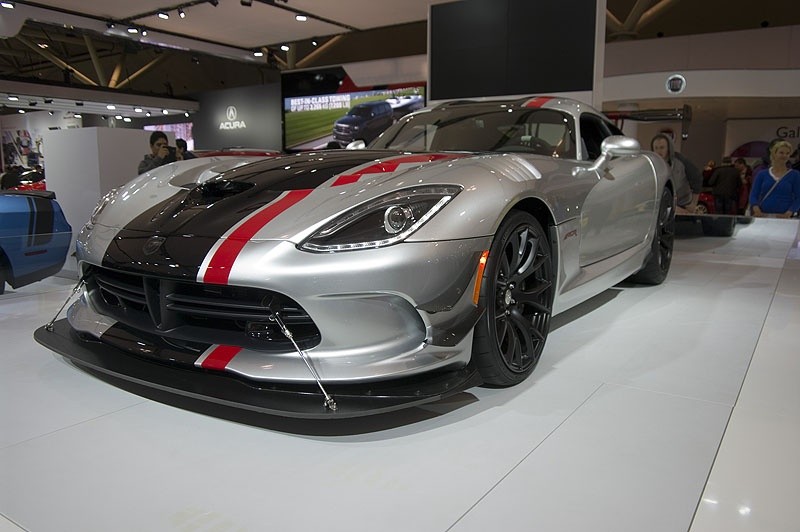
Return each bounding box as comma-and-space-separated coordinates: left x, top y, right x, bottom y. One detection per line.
0, 165, 73, 297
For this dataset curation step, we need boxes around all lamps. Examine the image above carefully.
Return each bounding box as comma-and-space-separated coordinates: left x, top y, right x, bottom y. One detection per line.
141, 28, 147, 36
178, 7, 186, 19
240, 0, 251, 6
0, 0, 15, 9
254, 48, 264, 57
158, 11, 169, 20
106, 22, 115, 29
128, 25, 139, 33
209, 0, 219, 7
8, 96, 84, 118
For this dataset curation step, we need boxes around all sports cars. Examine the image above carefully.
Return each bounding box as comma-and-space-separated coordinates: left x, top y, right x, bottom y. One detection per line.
33, 90, 685, 421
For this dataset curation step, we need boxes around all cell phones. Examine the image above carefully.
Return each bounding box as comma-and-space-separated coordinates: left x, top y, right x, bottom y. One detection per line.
163, 146, 176, 153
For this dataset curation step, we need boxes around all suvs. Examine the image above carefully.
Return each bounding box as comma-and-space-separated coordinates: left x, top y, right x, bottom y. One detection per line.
332, 100, 393, 148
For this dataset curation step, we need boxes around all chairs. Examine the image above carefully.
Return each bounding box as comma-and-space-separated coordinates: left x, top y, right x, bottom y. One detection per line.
525, 111, 571, 153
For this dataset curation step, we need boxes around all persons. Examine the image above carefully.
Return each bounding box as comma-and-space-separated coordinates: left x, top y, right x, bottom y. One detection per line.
650, 133, 696, 213
175, 138, 198, 161
137, 131, 183, 177
701, 156, 752, 215
0, 164, 42, 194
748, 141, 800, 220
